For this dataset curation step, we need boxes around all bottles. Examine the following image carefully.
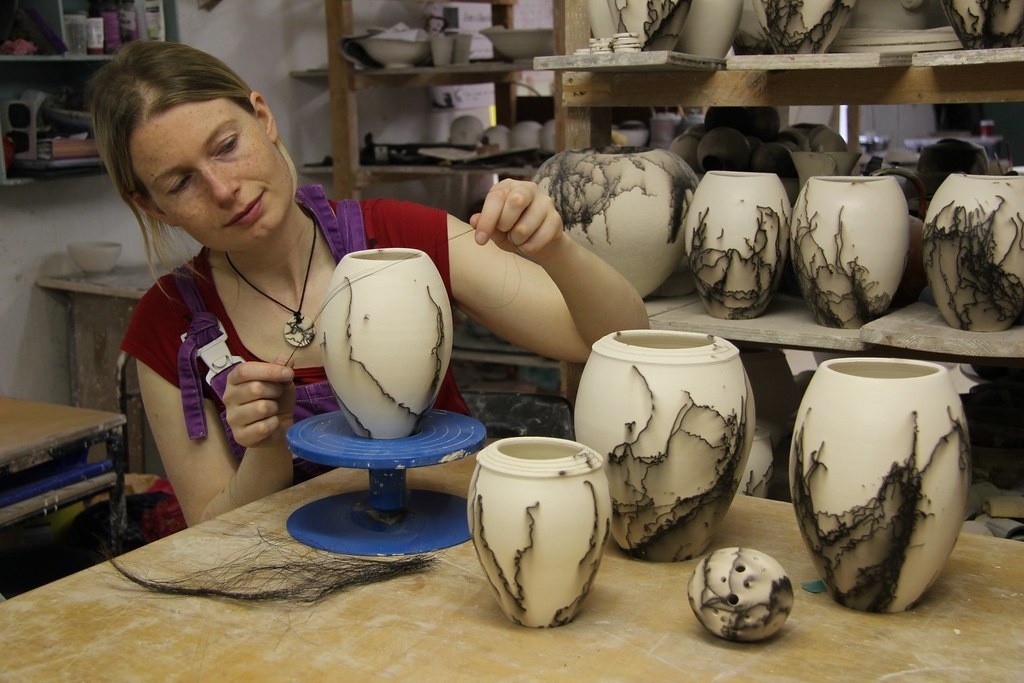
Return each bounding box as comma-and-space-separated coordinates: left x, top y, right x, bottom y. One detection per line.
320, 248, 453, 441
790, 355, 973, 612
586, 0, 1024, 52
574, 332, 756, 561
466, 435, 612, 627
98, 0, 140, 55
737, 424, 775, 499
533, 107, 1023, 331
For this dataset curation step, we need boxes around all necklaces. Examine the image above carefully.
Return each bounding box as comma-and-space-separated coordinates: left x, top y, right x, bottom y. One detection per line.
224, 207, 316, 347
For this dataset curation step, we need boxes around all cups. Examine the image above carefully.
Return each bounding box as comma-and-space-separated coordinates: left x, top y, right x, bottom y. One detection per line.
64, 14, 87, 55
429, 34, 472, 66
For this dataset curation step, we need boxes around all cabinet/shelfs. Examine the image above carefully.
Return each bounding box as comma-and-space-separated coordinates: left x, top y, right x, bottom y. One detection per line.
0, 0, 179, 186
290, 1, 559, 203
556, 1, 1024, 357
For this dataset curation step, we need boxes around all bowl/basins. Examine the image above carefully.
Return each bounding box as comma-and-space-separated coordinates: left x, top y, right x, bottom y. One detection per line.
67, 242, 123, 274
356, 38, 429, 69
479, 28, 555, 63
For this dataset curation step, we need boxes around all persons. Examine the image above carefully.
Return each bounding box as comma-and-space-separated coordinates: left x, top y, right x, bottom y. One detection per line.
86, 41, 651, 529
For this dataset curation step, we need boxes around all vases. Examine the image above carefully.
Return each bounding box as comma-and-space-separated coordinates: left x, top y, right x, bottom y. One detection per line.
320, 5, 1024, 636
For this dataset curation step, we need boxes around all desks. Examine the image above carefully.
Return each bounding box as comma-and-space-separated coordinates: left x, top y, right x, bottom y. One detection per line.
0, 448, 1023, 683
0, 394, 131, 598
37, 268, 170, 479
